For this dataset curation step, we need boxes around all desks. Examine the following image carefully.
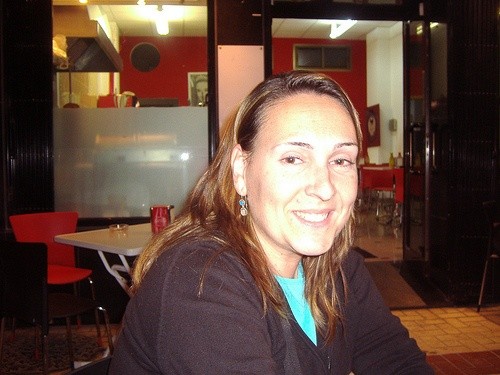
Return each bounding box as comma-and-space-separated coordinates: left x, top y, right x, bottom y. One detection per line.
53, 221, 161, 299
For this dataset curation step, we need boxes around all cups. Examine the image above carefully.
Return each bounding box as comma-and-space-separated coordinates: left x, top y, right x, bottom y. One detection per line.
150, 206, 170, 235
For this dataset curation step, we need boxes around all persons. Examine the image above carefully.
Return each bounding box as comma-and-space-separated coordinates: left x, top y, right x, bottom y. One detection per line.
109, 71, 435, 375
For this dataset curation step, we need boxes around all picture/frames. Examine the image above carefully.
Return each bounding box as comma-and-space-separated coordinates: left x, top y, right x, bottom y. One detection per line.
187, 72, 209, 105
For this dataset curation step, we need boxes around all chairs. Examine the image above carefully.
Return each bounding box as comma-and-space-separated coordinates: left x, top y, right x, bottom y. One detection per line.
0, 212, 116, 375
355, 167, 405, 230
476, 199, 500, 312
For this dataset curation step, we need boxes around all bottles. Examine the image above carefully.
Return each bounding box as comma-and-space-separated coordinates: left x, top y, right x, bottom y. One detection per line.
396, 152, 404, 168
389, 152, 395, 168
